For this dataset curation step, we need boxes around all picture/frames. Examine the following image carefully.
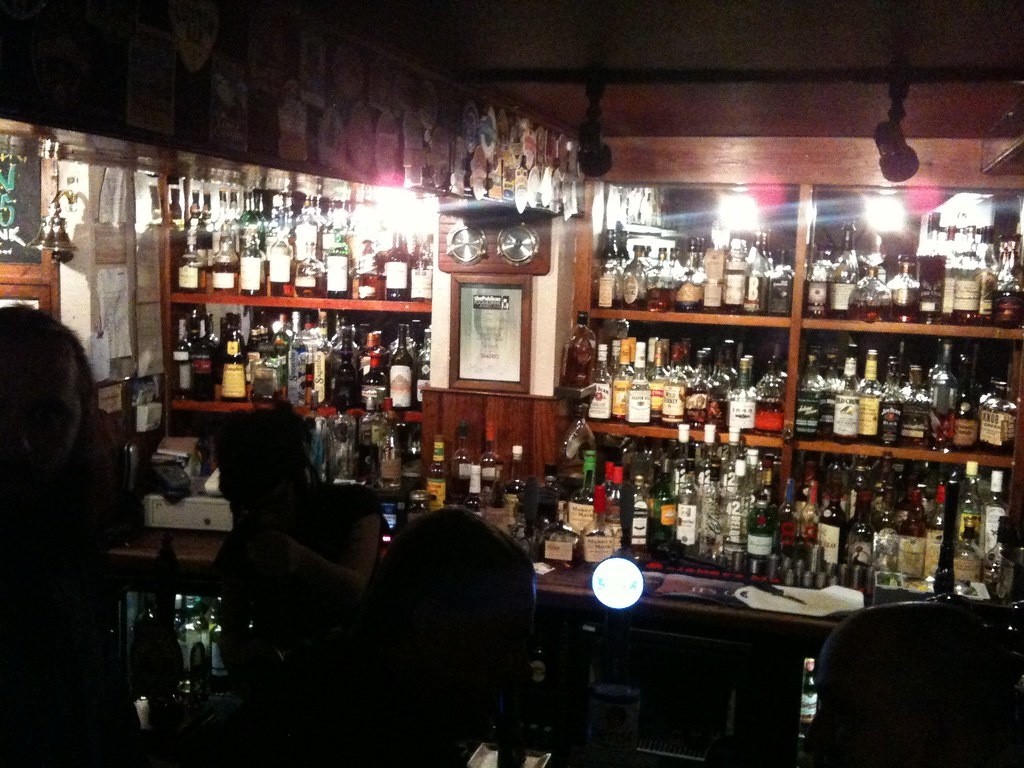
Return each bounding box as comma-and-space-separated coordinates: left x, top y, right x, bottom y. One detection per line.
449, 273, 532, 394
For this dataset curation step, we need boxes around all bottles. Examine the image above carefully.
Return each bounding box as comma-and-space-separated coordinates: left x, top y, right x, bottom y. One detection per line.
499, 689, 525, 768
171, 594, 227, 676
169, 189, 1024, 601
800, 657, 818, 723
133, 598, 156, 672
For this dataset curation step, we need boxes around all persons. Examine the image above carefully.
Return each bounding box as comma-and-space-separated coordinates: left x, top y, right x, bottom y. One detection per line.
208, 405, 388, 696
1, 302, 141, 768
800, 601, 1024, 768
179, 507, 540, 767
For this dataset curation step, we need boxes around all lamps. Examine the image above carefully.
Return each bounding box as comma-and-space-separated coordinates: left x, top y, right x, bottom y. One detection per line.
876, 80, 920, 181
576, 84, 611, 177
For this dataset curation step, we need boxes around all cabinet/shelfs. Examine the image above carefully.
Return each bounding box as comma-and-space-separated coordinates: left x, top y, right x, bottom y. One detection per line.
158, 137, 1024, 582
